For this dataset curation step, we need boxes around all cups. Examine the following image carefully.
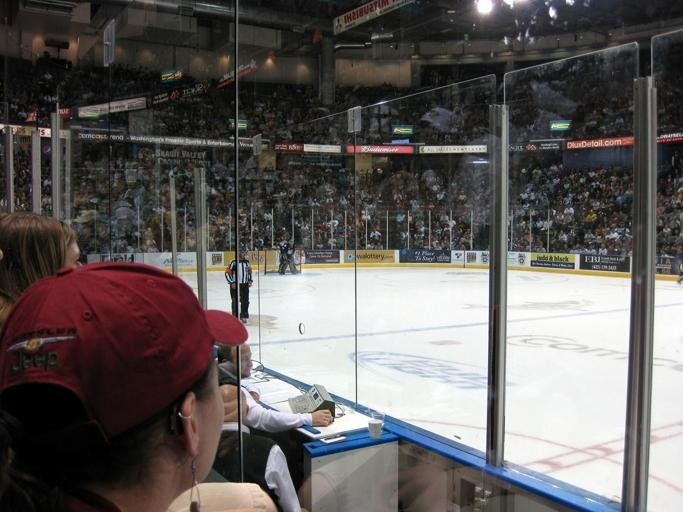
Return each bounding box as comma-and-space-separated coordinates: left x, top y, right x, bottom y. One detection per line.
365, 413, 384, 437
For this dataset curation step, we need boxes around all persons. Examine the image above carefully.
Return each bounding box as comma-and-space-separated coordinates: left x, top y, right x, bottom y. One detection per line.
0, 208, 88, 330
223, 246, 254, 325
0, 257, 283, 512
215, 340, 334, 439
212, 381, 301, 512
276, 232, 300, 275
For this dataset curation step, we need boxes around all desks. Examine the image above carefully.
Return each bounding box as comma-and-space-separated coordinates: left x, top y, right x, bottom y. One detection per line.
242, 366, 398, 512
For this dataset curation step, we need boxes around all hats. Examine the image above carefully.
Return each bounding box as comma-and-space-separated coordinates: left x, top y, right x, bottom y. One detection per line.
0, 258, 250, 463
